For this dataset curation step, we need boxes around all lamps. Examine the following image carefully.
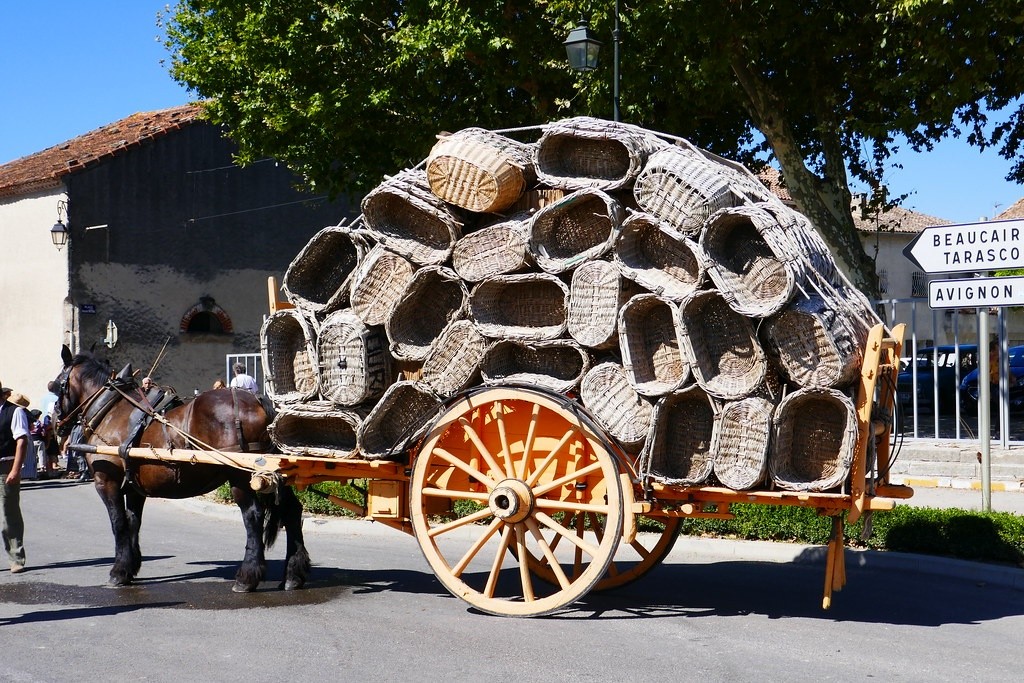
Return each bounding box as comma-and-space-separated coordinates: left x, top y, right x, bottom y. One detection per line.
50, 200, 69, 252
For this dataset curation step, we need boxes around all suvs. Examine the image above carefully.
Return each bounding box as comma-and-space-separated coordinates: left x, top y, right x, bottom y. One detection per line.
888, 343, 980, 417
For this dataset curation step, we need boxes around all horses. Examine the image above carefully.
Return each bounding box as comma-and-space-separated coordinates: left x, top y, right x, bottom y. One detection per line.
54, 341, 311, 593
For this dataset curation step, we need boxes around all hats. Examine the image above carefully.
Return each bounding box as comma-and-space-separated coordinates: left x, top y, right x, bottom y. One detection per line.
8, 393, 30, 409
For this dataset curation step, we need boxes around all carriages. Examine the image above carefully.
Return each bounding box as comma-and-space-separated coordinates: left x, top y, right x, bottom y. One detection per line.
47, 320, 912, 619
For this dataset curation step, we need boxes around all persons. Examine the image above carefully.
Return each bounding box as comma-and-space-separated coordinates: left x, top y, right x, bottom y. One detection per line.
231, 363, 260, 397
140, 377, 152, 394
989, 333, 1017, 439
3, 388, 94, 483
0, 382, 27, 572
214, 379, 226, 390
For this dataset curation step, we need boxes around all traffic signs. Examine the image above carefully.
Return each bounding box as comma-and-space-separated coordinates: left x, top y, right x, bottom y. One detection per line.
925, 278, 1023, 305
900, 220, 1023, 273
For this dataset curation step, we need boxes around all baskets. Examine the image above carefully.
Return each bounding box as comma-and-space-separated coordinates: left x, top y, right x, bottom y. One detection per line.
259, 122, 875, 491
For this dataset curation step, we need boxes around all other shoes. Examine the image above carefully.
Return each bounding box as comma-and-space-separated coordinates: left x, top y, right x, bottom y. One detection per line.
11, 561, 23, 573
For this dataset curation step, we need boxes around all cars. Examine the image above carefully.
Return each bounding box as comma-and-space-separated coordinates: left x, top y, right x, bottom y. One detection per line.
956, 344, 1024, 423
